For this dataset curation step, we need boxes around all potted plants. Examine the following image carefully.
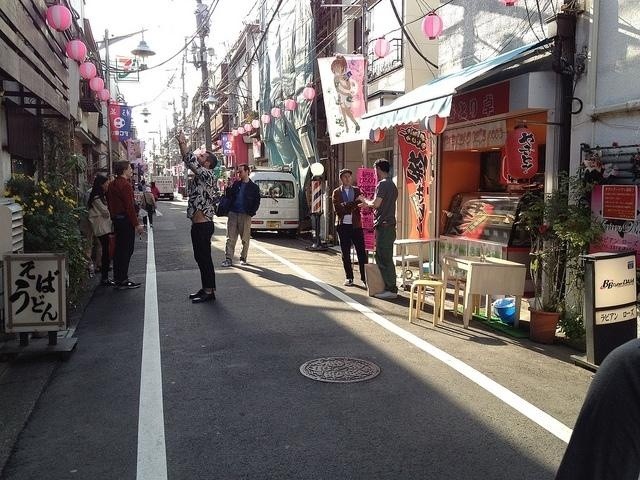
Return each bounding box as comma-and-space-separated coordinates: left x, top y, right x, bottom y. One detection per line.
513, 166, 607, 346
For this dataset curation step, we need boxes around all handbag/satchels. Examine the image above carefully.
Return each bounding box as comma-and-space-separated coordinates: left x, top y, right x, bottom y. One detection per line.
144, 202, 153, 216
216, 197, 229, 217
88, 198, 113, 237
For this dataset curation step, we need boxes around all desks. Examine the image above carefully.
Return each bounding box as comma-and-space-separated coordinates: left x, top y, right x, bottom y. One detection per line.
351, 238, 430, 294
437, 255, 528, 330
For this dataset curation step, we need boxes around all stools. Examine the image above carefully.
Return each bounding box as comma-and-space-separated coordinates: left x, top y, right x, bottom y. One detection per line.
392, 255, 423, 292
409, 278, 444, 327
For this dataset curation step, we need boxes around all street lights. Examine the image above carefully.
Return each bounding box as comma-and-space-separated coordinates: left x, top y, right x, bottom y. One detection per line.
104, 100, 154, 143
197, 94, 219, 167
95, 21, 157, 186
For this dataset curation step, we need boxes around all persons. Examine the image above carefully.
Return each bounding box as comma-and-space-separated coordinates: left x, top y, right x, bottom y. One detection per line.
332, 167, 370, 290
174, 128, 220, 303
221, 163, 261, 266
330, 55, 361, 134
86, 174, 161, 287
108, 159, 142, 290
354, 157, 399, 300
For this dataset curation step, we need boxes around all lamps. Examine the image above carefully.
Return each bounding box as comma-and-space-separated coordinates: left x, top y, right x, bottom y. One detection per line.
96, 25, 155, 56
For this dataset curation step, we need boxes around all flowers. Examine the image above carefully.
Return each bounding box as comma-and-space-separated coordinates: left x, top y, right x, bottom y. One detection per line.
37, 172, 81, 212
0, 172, 54, 216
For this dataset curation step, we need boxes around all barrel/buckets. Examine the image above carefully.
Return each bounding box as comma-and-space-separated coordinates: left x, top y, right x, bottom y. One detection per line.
492, 297, 516, 325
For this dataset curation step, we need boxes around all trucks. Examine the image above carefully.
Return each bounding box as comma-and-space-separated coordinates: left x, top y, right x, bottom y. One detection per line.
148, 175, 176, 200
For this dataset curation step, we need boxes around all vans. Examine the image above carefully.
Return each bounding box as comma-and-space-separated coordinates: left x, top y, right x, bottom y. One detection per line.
235, 165, 303, 238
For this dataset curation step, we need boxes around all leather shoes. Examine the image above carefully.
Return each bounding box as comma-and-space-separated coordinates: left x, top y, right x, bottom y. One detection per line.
189, 288, 216, 304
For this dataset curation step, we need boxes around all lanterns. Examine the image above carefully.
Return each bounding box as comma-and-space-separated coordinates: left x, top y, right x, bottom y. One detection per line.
506, 123, 539, 180
423, 13, 444, 41
97, 88, 111, 102
425, 113, 449, 136
285, 98, 297, 111
231, 119, 261, 137
68, 39, 88, 62
303, 87, 316, 99
79, 62, 97, 79
88, 77, 105, 92
369, 127, 386, 144
271, 107, 282, 118
374, 38, 391, 58
47, 4, 73, 30
261, 114, 271, 124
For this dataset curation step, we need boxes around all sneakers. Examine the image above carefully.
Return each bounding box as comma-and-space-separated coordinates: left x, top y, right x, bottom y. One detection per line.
96, 267, 142, 290
343, 277, 398, 299
221, 255, 248, 267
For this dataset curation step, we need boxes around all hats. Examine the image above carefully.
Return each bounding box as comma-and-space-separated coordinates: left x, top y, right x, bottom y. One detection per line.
145, 187, 152, 192
339, 169, 352, 176
